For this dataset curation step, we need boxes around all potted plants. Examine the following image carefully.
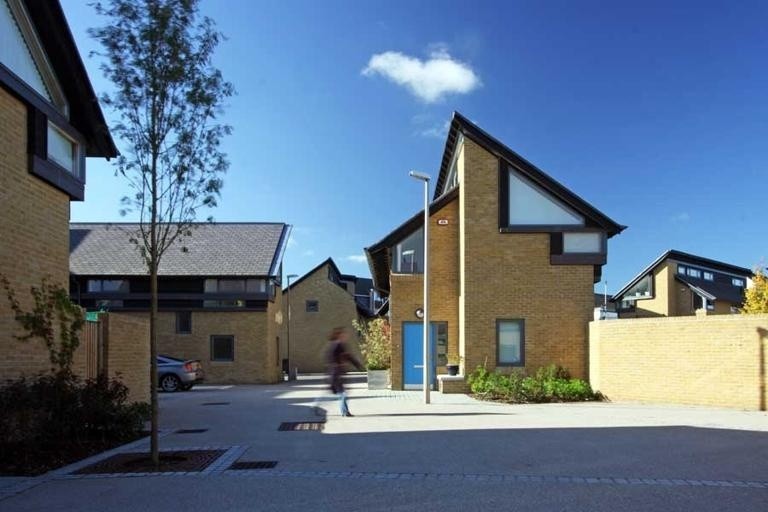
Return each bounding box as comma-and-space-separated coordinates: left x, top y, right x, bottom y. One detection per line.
445, 353, 461, 375
351, 318, 391, 390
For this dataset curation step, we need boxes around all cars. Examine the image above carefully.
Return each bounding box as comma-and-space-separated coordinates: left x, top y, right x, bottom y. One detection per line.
155, 352, 204, 392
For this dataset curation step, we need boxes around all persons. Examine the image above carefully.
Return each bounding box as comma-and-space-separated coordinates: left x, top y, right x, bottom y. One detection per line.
311, 325, 353, 419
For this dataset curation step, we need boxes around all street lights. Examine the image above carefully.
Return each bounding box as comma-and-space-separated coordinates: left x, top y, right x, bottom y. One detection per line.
286, 274, 301, 375
408, 171, 432, 403
